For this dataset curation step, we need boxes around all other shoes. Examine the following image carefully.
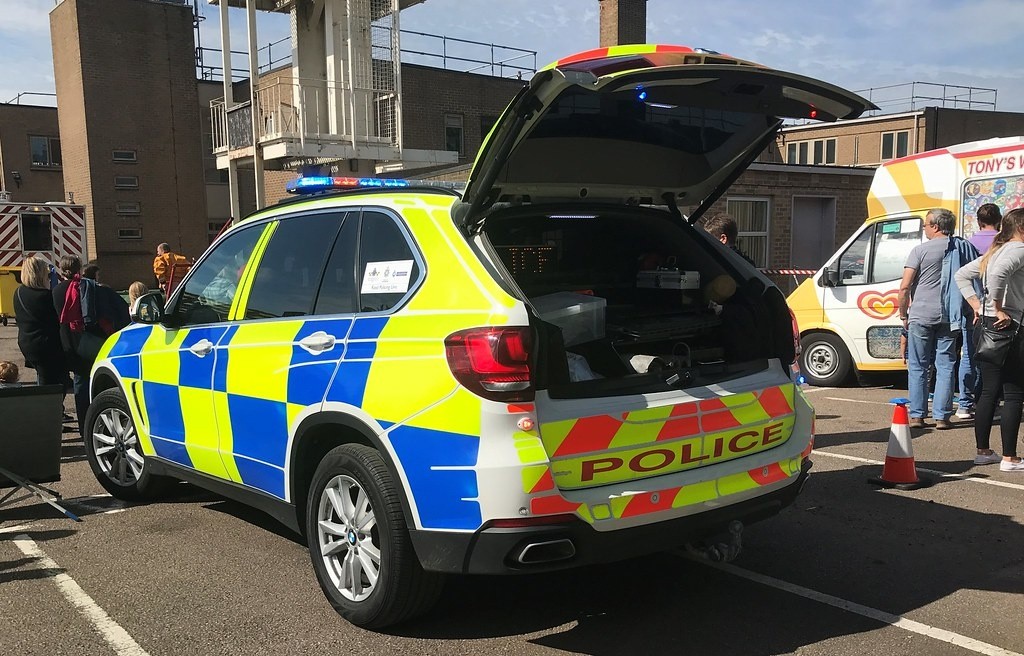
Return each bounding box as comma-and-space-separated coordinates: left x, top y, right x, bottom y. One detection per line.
62, 413, 74, 422
1000, 457, 1024, 472
936, 419, 954, 429
955, 407, 972, 419
927, 393, 934, 402
952, 396, 960, 405
909, 417, 924, 427
974, 449, 1003, 465
61, 425, 73, 433
993, 408, 1002, 420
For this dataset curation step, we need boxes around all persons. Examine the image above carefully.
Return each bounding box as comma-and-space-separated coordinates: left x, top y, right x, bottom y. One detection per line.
954, 203, 1002, 418
198, 261, 248, 307
901, 314, 908, 365
129, 282, 157, 316
953, 208, 1024, 472
0, 361, 18, 383
52, 254, 132, 437
13, 257, 74, 432
705, 213, 755, 267
898, 208, 980, 428
153, 242, 191, 293
82, 264, 99, 282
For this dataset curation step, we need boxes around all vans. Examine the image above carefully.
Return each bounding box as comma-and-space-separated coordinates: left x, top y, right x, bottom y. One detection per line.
786, 134, 1023, 389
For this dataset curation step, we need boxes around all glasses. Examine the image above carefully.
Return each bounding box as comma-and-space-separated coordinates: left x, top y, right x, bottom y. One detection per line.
921, 222, 941, 230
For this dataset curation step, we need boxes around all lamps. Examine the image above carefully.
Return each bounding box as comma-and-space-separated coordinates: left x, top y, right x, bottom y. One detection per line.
11, 171, 22, 189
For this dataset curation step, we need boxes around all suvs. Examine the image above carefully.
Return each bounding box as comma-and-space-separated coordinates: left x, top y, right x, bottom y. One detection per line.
82, 43, 882, 633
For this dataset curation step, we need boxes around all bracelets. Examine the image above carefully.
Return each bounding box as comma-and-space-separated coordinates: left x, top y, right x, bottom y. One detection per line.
900, 316, 907, 320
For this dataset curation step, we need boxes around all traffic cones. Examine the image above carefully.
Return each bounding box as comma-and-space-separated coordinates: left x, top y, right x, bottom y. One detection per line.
868, 404, 933, 492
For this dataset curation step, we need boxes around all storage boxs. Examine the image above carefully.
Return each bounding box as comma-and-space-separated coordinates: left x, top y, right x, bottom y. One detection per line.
607, 304, 724, 355
527, 291, 607, 347
636, 270, 700, 290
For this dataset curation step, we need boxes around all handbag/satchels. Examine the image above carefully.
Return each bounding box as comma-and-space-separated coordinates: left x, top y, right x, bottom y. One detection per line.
972, 326, 1016, 367
75, 332, 106, 361
